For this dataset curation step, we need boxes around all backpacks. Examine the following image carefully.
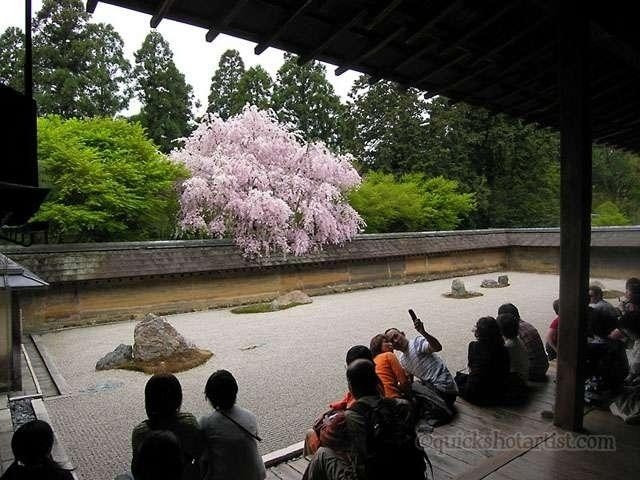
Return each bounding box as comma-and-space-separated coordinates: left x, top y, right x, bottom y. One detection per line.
346, 398, 434, 480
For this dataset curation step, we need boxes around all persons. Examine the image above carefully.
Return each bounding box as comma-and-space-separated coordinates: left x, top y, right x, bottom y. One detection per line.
133, 373, 199, 480
302, 359, 426, 479
304, 277, 639, 457
3, 420, 75, 479
198, 370, 266, 480
114, 431, 194, 480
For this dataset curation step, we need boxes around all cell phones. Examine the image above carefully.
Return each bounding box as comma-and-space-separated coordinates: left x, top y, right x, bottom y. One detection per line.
408, 309, 417, 322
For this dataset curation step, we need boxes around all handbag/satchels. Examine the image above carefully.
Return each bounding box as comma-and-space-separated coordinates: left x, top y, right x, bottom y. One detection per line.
455, 372, 483, 405
